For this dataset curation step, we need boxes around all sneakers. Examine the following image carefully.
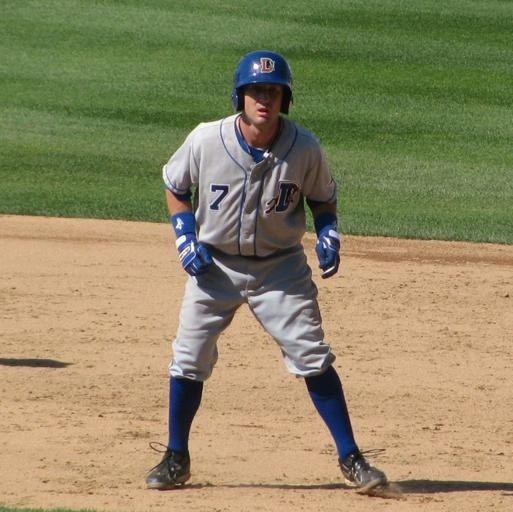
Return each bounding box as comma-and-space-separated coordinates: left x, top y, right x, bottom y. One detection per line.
144, 447, 191, 490
335, 450, 388, 493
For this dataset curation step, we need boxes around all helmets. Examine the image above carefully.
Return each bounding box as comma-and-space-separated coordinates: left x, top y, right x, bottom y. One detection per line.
228, 50, 296, 116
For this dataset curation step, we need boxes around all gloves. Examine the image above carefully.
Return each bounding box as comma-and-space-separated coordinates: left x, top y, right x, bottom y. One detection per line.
310, 213, 341, 281
169, 210, 212, 279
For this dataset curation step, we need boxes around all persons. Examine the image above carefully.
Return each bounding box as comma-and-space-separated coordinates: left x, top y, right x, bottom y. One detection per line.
146, 50, 387, 495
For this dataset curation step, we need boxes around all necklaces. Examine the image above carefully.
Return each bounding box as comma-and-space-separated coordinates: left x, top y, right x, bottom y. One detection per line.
237, 116, 281, 160
